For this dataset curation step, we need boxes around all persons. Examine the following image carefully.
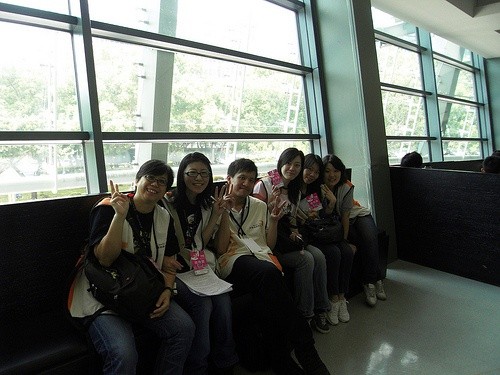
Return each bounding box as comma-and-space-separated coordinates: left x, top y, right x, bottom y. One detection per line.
297, 153, 353, 325
213, 158, 331, 375
481, 157, 500, 174
321, 154, 387, 306
67, 159, 194, 375
492, 150, 500, 157
162, 152, 251, 374
252, 147, 330, 334
401, 151, 424, 168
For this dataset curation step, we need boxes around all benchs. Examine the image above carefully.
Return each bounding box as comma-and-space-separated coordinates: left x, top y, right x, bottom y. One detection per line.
0, 182, 255, 375
388, 168, 500, 286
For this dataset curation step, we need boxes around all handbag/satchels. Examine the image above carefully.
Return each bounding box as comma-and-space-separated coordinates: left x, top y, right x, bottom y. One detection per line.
302, 215, 344, 245
277, 215, 303, 255
83, 237, 166, 329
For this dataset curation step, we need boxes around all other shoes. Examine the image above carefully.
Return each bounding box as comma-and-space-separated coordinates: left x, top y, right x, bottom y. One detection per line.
293, 338, 332, 375
272, 353, 305, 375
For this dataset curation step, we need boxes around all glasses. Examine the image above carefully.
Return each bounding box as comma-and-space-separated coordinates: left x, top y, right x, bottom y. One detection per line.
142, 174, 169, 186
183, 171, 211, 178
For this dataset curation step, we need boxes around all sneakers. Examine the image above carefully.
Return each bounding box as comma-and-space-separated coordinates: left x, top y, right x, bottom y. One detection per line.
362, 282, 377, 306
326, 297, 341, 325
375, 279, 387, 300
313, 308, 330, 334
337, 297, 351, 323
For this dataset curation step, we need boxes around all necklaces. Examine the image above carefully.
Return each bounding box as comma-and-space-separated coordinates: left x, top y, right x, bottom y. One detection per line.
282, 187, 289, 190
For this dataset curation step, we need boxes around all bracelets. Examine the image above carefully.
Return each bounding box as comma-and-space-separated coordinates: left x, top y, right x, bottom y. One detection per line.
162, 287, 174, 296
